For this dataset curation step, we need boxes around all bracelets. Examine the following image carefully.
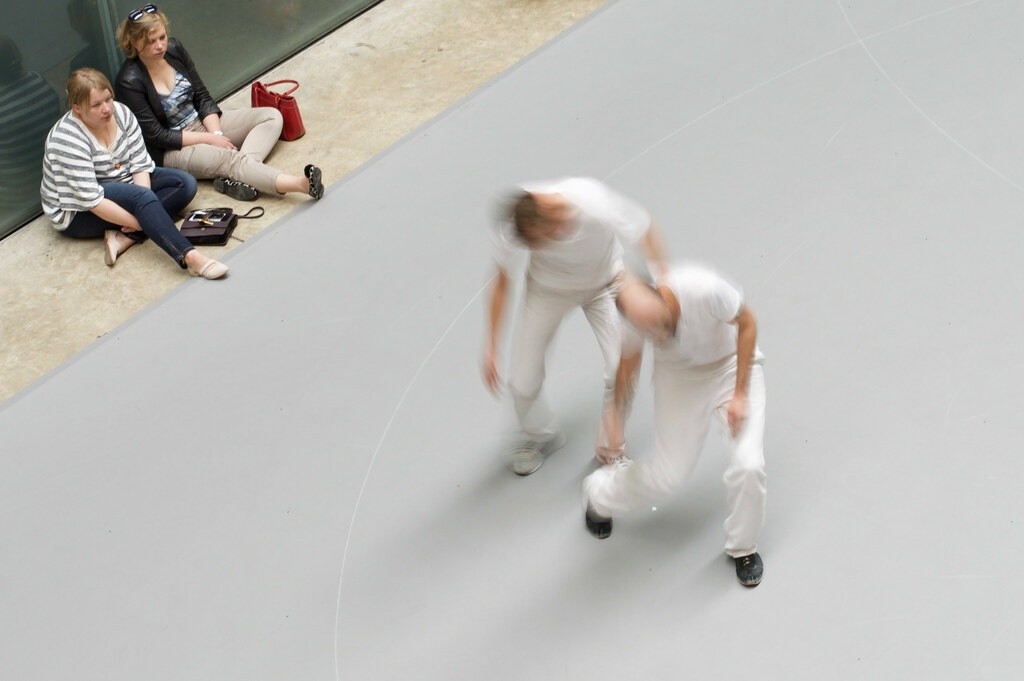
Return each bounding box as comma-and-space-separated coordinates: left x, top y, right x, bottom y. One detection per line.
214, 131, 222, 135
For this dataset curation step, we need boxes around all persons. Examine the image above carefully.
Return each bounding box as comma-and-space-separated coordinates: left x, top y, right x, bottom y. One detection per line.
583, 266, 765, 585
40, 68, 228, 280
481, 178, 668, 478
114, 4, 324, 201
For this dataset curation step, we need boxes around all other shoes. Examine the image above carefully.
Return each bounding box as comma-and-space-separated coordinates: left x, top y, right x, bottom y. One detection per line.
213, 177, 260, 201
105, 230, 121, 266
187, 259, 230, 279
304, 164, 324, 200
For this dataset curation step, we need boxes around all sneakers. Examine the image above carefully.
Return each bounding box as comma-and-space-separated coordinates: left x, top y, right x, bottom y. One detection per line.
735, 552, 764, 585
586, 498, 614, 539
512, 435, 558, 475
595, 453, 632, 470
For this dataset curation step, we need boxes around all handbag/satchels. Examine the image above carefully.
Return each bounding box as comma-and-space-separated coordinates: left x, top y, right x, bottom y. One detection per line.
180, 208, 236, 246
251, 79, 305, 141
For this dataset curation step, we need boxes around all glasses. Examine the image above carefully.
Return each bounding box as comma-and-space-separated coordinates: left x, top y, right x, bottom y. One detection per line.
128, 3, 158, 29
